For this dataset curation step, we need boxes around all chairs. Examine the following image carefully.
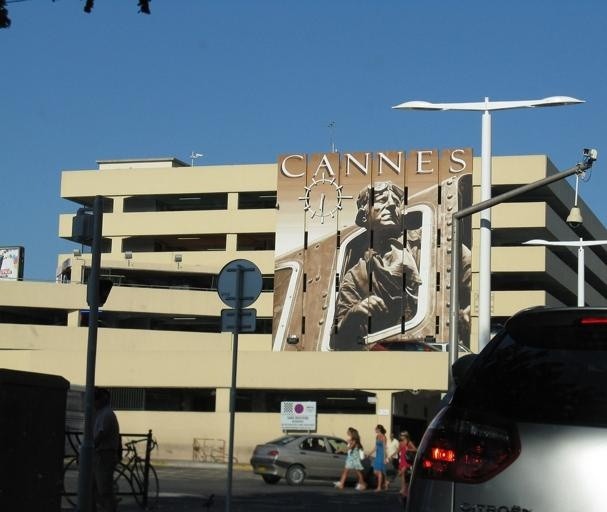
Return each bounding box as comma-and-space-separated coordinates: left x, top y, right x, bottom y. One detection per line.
355, 484, 366, 490
332, 481, 343, 489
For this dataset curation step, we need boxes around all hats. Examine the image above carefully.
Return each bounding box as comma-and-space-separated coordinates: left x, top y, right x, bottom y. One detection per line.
397, 304, 607, 512
249, 432, 399, 488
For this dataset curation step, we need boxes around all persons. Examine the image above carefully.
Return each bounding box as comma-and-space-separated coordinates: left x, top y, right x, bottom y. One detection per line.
385, 429, 399, 476
386, 431, 417, 496
366, 424, 390, 493
329, 427, 367, 491
328, 178, 471, 351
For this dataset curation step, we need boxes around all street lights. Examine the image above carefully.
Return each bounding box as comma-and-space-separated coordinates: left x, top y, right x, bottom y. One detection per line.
404, 440, 415, 464
348, 449, 365, 461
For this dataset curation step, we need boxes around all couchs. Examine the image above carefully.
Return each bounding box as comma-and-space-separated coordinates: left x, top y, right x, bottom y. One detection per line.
86, 276, 113, 307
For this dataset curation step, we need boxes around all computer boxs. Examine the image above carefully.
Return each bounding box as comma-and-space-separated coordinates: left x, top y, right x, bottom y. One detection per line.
566, 207, 582, 228
582, 148, 598, 160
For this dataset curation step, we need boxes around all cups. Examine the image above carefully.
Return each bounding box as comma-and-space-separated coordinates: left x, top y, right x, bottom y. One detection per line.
367, 182, 404, 198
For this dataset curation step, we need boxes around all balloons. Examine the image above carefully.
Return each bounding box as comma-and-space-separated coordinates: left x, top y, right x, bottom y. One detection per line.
296, 172, 352, 224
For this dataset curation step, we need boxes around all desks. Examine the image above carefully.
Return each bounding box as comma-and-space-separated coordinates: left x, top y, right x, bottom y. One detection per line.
520, 236, 607, 309
447, 146, 601, 401
388, 92, 590, 360
